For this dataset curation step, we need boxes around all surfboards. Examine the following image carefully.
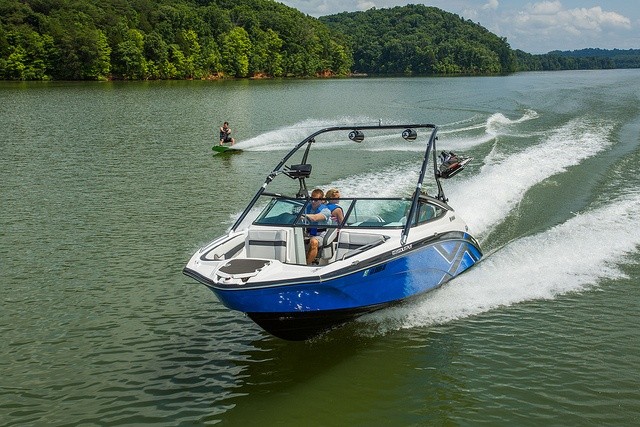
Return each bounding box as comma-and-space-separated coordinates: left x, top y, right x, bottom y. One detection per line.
212, 145, 243, 153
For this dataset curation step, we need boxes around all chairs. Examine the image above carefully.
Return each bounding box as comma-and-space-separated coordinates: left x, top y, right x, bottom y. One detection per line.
371, 216, 383, 223
419, 202, 436, 223
305, 220, 339, 265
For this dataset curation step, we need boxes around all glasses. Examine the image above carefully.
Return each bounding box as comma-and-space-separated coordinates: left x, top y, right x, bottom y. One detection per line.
309, 197, 318, 201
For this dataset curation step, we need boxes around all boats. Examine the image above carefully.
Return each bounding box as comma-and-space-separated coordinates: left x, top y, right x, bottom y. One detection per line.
183, 119, 483, 341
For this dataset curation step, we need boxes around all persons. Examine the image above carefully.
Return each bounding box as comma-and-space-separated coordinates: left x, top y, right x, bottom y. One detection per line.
219, 122, 234, 146
302, 189, 332, 264
325, 189, 345, 225
